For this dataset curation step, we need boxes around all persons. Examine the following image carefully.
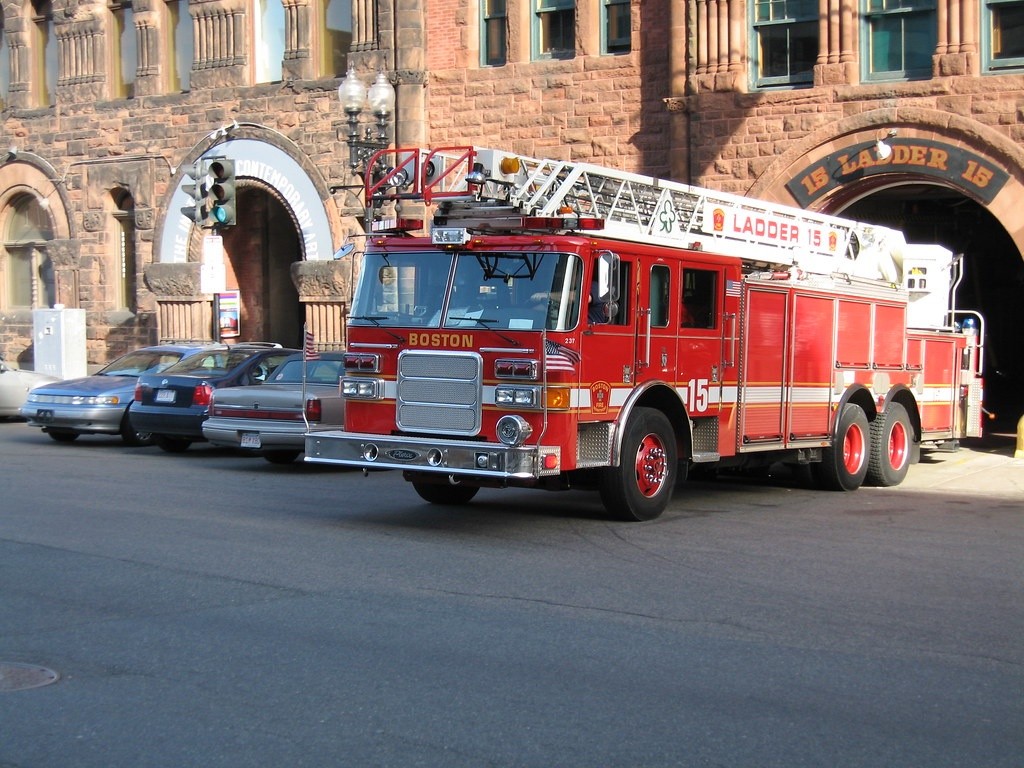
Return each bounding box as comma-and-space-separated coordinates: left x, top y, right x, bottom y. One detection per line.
531, 271, 606, 329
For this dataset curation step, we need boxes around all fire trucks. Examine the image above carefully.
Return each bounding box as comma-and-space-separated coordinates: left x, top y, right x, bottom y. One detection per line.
302, 144, 984, 521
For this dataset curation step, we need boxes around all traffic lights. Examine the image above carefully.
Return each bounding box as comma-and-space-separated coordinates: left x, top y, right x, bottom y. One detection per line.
209, 160, 237, 227
180, 160, 206, 227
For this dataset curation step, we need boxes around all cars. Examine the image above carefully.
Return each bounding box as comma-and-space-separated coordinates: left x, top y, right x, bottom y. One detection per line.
128, 346, 304, 451
201, 352, 345, 463
19, 341, 283, 446
0, 357, 65, 419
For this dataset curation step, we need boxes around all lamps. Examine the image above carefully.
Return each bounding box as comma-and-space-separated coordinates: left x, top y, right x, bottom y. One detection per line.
875, 128, 898, 161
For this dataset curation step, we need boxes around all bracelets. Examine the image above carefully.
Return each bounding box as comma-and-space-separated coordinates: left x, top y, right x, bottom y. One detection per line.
544, 291, 550, 299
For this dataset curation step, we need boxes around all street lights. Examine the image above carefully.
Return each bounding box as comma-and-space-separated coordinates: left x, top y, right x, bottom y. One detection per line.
328, 63, 397, 231
